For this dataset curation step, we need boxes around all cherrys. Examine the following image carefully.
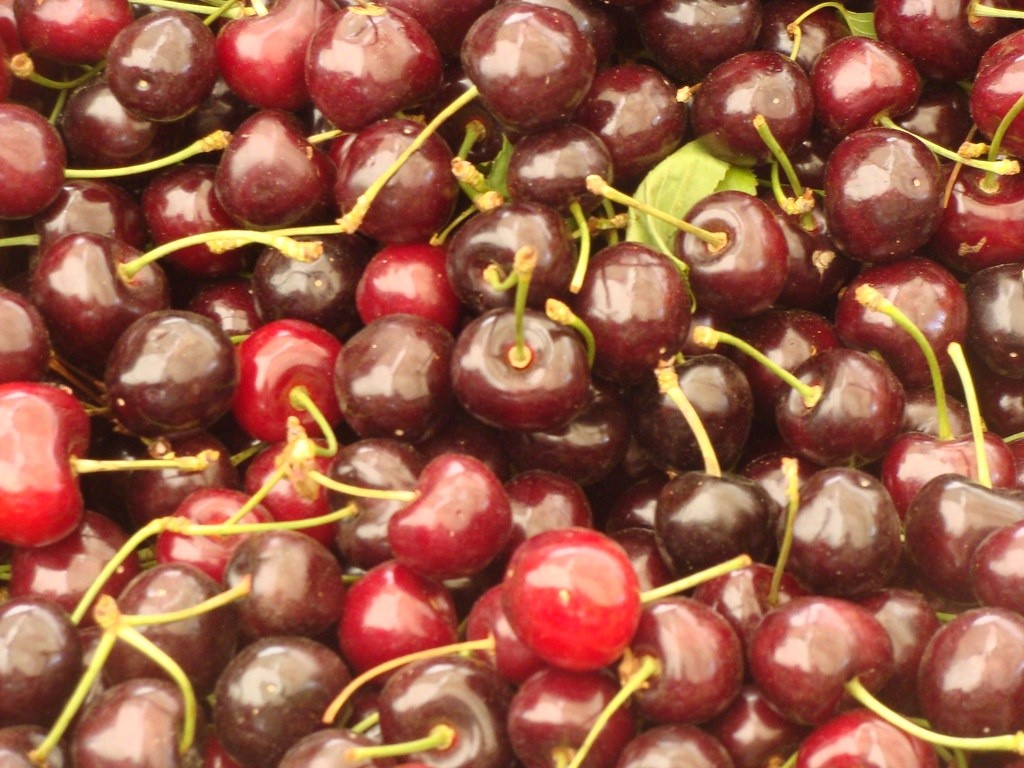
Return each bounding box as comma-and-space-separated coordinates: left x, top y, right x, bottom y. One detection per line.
1, 0, 1024, 768
